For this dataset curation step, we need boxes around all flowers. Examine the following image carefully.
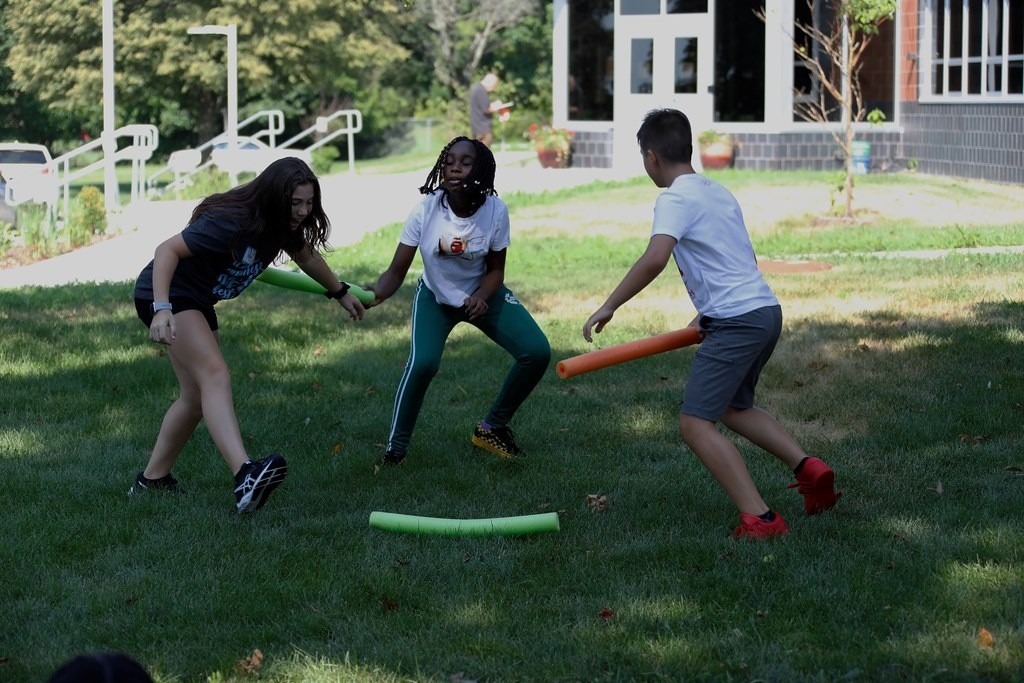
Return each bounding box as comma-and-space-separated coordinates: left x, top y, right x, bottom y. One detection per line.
528, 125, 575, 153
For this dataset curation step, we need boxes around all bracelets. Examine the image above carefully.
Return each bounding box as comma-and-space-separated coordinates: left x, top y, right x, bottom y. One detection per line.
324, 282, 350, 299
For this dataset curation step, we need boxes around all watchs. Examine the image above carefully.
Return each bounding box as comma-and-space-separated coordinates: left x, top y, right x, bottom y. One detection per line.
150, 302, 172, 316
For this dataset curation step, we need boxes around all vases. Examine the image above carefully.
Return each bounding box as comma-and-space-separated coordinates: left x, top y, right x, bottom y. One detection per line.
536, 146, 571, 169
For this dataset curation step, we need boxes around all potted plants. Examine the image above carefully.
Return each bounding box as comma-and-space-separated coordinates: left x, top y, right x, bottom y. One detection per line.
699, 130, 733, 168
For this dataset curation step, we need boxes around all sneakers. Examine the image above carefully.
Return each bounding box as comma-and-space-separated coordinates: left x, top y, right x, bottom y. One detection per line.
472, 422, 527, 459
128, 472, 195, 499
233, 456, 287, 516
788, 457, 836, 518
729, 513, 789, 542
376, 453, 405, 470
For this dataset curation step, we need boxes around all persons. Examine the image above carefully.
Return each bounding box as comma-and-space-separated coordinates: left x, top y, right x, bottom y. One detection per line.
356, 136, 552, 466
583, 108, 843, 541
128, 157, 364, 514
469, 73, 502, 147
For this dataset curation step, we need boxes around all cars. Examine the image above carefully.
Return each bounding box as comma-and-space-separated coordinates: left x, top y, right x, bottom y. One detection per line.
0, 139, 58, 205
168, 136, 312, 174
